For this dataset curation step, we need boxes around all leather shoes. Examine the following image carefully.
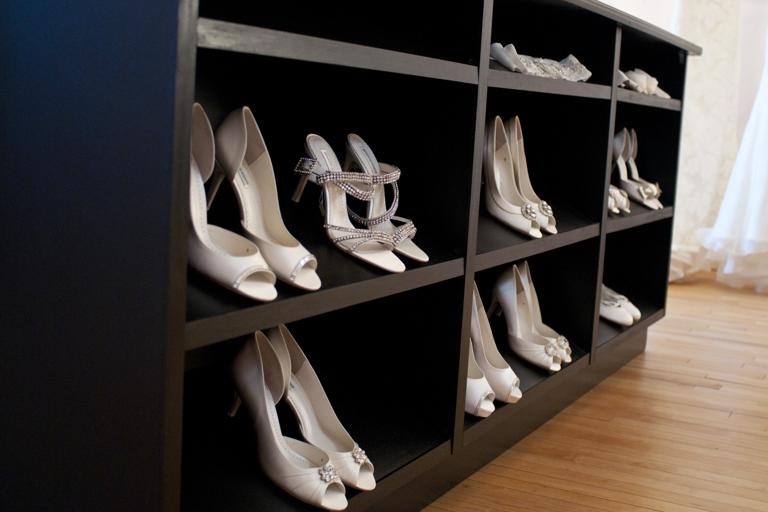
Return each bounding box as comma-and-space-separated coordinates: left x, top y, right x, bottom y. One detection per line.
599, 283, 642, 326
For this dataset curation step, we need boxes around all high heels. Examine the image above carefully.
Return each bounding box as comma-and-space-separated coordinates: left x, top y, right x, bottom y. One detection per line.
207, 105, 322, 290
483, 115, 558, 239
465, 261, 572, 418
228, 323, 376, 512
293, 133, 406, 273
343, 133, 430, 263
188, 103, 278, 302
608, 127, 664, 214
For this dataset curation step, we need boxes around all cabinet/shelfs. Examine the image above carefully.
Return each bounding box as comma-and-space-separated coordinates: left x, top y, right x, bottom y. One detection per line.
1, 0, 708, 512
